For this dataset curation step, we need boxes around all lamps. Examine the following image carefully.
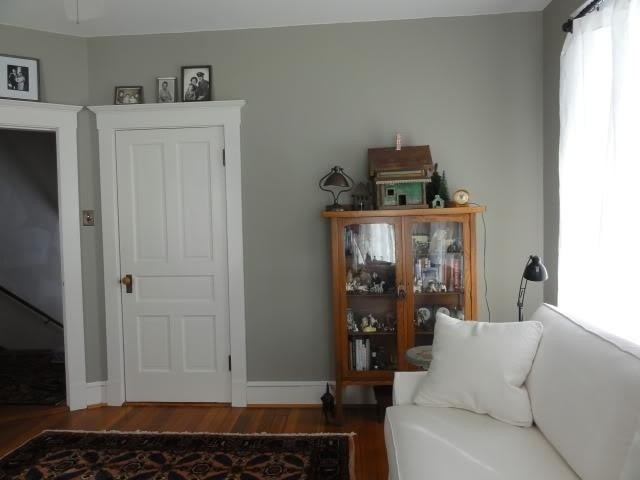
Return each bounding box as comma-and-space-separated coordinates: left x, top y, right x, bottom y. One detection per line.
318, 163, 356, 211
513, 251, 548, 323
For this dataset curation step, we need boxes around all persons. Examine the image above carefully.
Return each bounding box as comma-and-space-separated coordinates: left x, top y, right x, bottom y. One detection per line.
119, 90, 141, 104
196, 72, 210, 100
184, 77, 200, 101
8, 67, 25, 90
159, 82, 172, 102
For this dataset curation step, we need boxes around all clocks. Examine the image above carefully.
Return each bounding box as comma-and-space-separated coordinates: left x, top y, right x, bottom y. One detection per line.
453, 188, 472, 207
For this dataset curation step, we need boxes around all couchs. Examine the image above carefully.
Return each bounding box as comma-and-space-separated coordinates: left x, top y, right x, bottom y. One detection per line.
384, 299, 640, 480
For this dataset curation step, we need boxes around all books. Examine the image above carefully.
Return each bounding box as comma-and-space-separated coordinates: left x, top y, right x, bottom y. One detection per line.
349, 336, 373, 371
444, 257, 463, 291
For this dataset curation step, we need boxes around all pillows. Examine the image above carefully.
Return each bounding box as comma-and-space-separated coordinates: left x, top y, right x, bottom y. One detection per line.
409, 308, 546, 432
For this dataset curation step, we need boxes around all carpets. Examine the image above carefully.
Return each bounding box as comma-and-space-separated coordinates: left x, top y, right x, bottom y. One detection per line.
1, 428, 359, 479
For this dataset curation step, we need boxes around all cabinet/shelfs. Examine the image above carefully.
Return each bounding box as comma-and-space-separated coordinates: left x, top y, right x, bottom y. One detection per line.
322, 202, 485, 424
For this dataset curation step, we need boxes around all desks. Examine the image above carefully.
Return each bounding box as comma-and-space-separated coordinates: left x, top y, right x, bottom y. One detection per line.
405, 344, 433, 370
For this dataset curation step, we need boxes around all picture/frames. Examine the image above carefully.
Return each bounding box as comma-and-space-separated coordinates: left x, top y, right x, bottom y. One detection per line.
114, 84, 145, 105
0, 53, 40, 101
181, 64, 214, 102
155, 75, 178, 104
